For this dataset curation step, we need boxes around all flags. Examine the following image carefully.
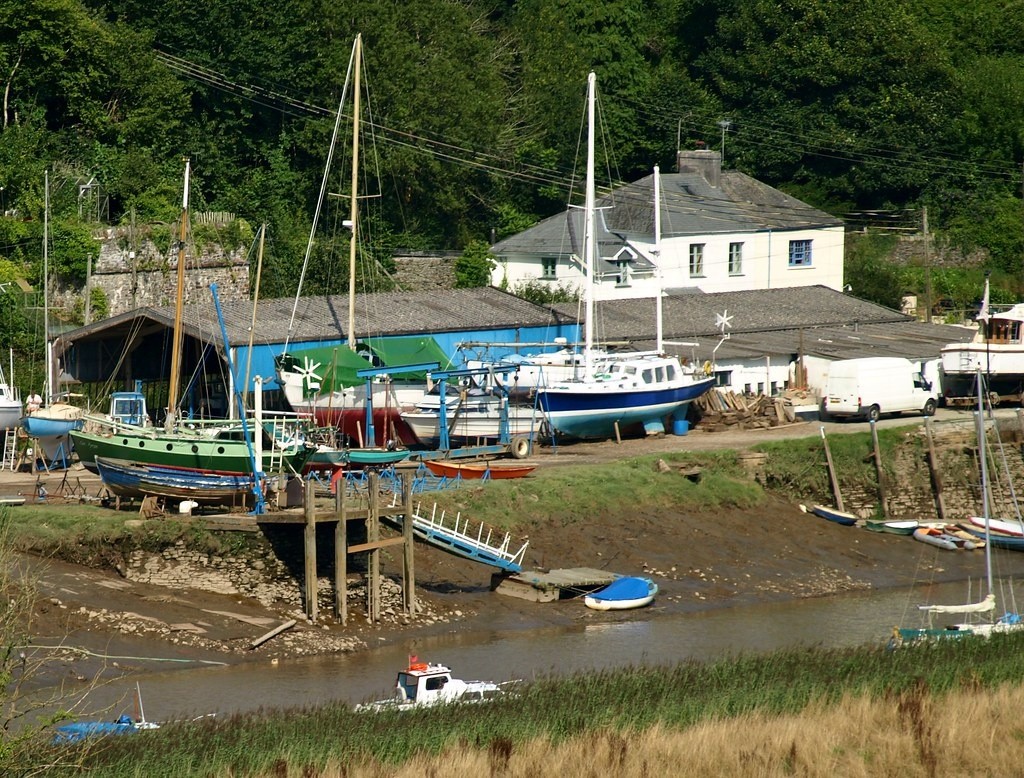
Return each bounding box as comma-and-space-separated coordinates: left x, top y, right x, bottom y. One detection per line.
408, 654, 419, 663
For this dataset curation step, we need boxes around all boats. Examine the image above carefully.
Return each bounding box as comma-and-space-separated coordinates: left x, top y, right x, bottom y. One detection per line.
866, 520, 918, 536
914, 527, 975, 551
812, 505, 859, 527
585, 574, 659, 613
354, 652, 505, 714
423, 457, 537, 478
940, 270, 1024, 396
94, 455, 268, 505
0, 360, 23, 430
943, 525, 987, 548
954, 516, 1024, 551
52, 680, 161, 745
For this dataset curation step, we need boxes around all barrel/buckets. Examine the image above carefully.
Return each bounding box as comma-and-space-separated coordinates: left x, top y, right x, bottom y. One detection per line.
674, 420, 688, 436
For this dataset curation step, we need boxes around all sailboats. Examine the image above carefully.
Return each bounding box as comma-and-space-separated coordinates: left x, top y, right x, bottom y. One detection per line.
887, 361, 1024, 651
263, 32, 717, 473
69, 161, 318, 479
19, 170, 86, 440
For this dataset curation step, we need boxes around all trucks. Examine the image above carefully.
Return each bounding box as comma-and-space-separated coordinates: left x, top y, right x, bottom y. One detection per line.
820, 357, 938, 422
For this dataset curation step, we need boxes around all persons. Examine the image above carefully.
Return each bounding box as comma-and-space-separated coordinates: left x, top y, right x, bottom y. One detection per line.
17, 424, 29, 456
26, 389, 42, 414
